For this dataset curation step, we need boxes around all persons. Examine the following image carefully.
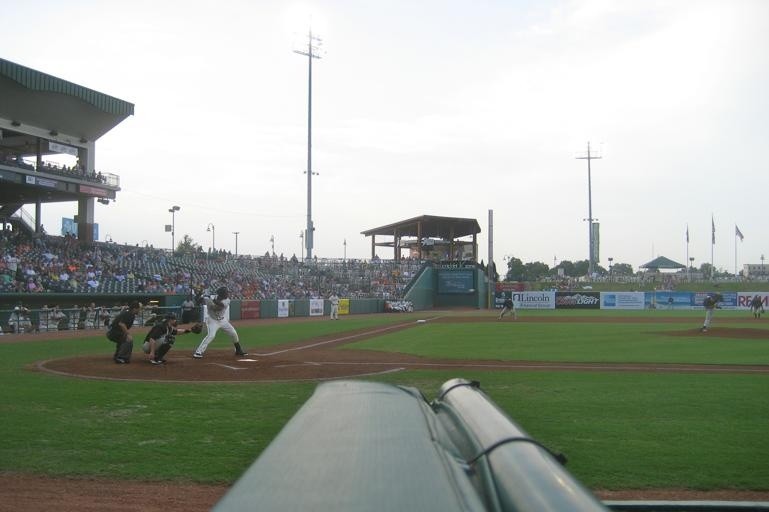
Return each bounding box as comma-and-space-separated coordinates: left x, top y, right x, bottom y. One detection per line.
701, 293, 721, 333
750, 294, 764, 320
247, 251, 462, 312
193, 286, 248, 358
3, 152, 104, 184
106, 302, 141, 366
328, 292, 340, 320
1, 224, 249, 331
142, 314, 204, 364
498, 296, 517, 320
461, 252, 752, 291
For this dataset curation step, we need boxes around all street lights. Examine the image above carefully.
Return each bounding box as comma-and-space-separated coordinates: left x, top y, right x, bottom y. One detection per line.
690, 258, 695, 281
607, 258, 613, 275
760, 256, 764, 272
208, 223, 214, 253
169, 206, 180, 254
234, 231, 239, 255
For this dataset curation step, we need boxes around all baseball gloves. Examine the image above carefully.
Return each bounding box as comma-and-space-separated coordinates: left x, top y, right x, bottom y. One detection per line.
191, 323, 203, 333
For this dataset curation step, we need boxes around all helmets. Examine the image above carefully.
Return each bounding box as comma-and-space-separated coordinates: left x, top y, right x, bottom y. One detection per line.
165, 314, 177, 327
217, 287, 228, 299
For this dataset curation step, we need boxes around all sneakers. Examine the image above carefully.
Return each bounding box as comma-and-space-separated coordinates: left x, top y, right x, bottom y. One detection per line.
158, 360, 166, 363
194, 354, 202, 357
117, 358, 129, 364
149, 359, 158, 366
236, 351, 248, 356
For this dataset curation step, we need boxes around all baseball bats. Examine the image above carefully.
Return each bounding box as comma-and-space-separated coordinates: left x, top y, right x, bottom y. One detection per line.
205, 267, 215, 294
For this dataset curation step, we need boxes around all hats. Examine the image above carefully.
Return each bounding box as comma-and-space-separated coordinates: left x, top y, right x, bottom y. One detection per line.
130, 300, 142, 309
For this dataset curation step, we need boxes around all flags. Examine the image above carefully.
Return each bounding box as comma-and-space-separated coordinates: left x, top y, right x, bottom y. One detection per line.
711, 217, 715, 245
685, 226, 690, 243
735, 225, 743, 240
